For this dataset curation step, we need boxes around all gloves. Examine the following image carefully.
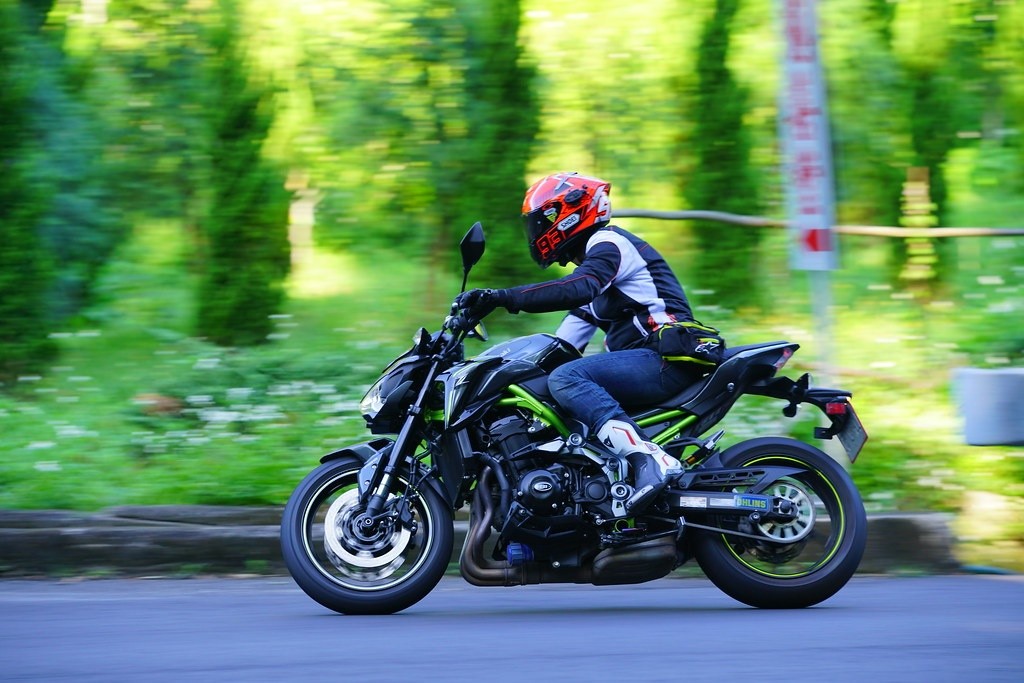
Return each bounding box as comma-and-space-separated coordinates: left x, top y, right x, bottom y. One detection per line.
457, 287, 503, 328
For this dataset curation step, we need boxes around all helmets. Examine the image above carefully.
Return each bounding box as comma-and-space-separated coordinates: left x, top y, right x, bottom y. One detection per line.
520, 172, 610, 269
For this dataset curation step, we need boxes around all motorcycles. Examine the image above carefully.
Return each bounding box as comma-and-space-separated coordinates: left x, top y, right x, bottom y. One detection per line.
280, 222, 869, 619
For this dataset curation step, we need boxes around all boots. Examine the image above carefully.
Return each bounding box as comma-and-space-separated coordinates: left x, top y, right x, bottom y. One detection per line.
595, 412, 685, 504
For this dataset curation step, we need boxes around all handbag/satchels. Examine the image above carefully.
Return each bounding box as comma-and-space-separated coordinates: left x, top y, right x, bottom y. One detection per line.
645, 317, 728, 372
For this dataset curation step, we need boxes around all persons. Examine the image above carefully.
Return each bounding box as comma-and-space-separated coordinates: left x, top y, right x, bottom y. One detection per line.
450, 172, 703, 510
